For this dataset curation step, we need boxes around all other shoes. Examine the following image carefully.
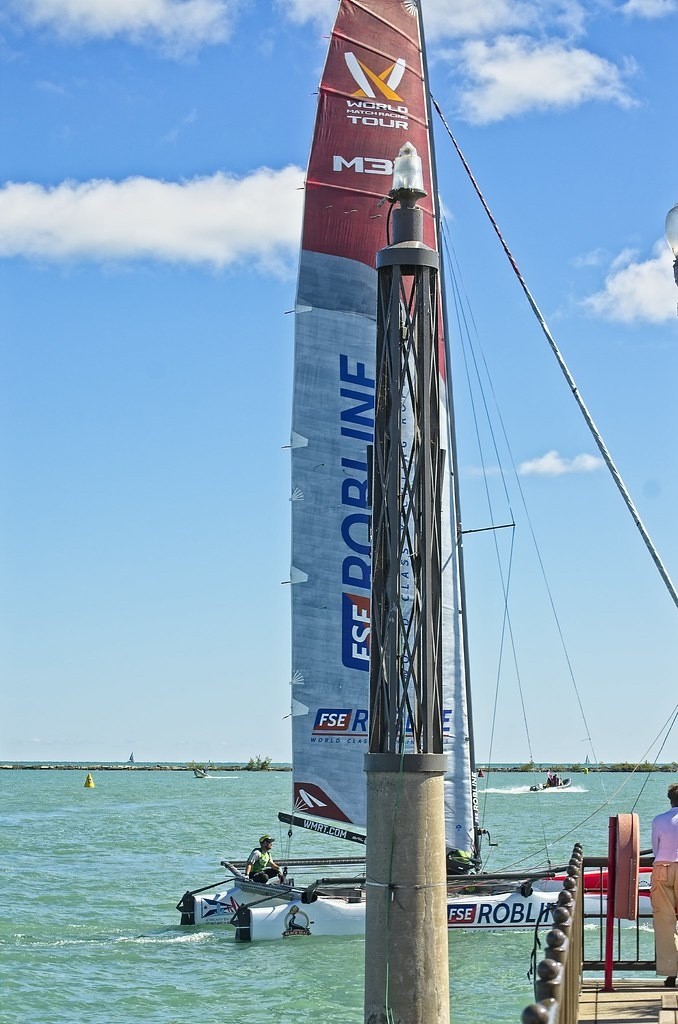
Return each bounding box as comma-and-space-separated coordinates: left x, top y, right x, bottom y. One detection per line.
664, 977, 678, 987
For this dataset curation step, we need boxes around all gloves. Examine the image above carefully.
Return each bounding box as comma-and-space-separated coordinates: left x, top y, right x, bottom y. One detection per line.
244, 876, 249, 881
277, 867, 283, 876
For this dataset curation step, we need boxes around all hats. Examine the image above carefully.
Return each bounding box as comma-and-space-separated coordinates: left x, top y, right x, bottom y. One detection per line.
259, 835, 275, 846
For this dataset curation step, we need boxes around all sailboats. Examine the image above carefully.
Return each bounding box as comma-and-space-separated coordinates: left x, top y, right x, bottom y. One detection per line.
171, 1, 580, 940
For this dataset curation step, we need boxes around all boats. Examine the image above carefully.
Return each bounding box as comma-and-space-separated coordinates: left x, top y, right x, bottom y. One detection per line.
530, 778, 572, 793
194, 769, 208, 779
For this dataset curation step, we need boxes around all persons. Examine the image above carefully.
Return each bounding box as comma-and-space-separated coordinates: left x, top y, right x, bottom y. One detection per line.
651, 782, 678, 987
547, 768, 563, 787
246, 835, 283, 884
